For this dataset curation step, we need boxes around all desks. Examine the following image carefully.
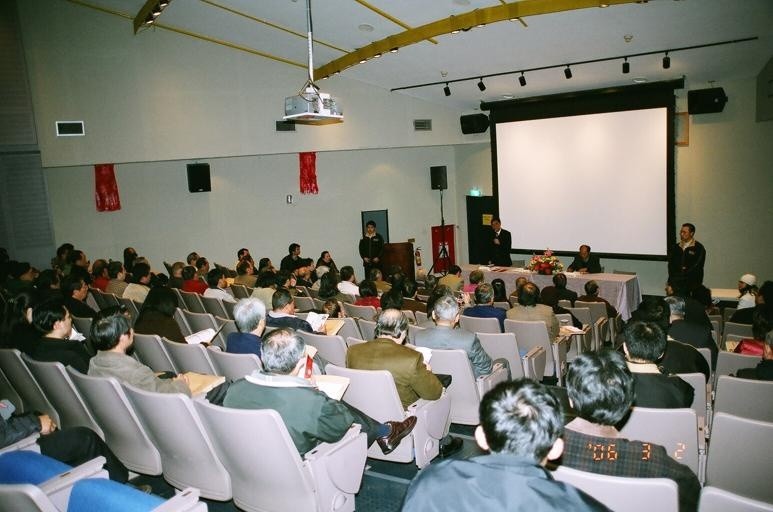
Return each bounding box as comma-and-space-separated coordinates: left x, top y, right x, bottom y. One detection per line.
383, 243, 415, 283
709, 288, 765, 304
463, 265, 642, 324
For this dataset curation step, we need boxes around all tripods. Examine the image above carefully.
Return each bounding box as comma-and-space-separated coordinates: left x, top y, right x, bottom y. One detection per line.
427, 190, 454, 275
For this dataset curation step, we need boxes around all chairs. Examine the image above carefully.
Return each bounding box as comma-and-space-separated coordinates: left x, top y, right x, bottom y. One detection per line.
558, 300, 571, 307
407, 324, 425, 345
562, 308, 609, 352
341, 293, 354, 304
296, 329, 347, 368
293, 296, 316, 311
170, 288, 188, 311
1, 456, 208, 512
347, 337, 367, 347
358, 319, 376, 340
133, 333, 179, 376
512, 259, 525, 267
708, 315, 722, 329
417, 294, 430, 302
404, 343, 508, 425
121, 380, 232, 501
115, 295, 139, 316
415, 311, 427, 325
261, 326, 279, 337
198, 294, 228, 319
0, 433, 109, 512
20, 352, 105, 442
668, 373, 714, 438
377, 307, 416, 325
308, 287, 319, 298
706, 412, 773, 505
723, 307, 736, 322
715, 351, 762, 391
193, 398, 367, 512
295, 286, 311, 297
65, 365, 162, 476
551, 466, 679, 512
198, 276, 209, 285
711, 330, 722, 350
0, 368, 24, 414
222, 299, 238, 320
710, 321, 722, 334
161, 337, 217, 376
726, 334, 753, 341
554, 314, 592, 363
313, 298, 344, 318
698, 487, 773, 512
246, 286, 253, 297
493, 302, 510, 310
0, 432, 40, 456
100, 292, 120, 307
694, 348, 716, 383
510, 296, 518, 308
574, 300, 622, 349
178, 289, 206, 313
504, 319, 567, 387
324, 363, 451, 470
70, 314, 93, 339
459, 315, 501, 333
207, 346, 265, 382
174, 307, 192, 337
327, 317, 363, 343
714, 375, 773, 422
295, 313, 309, 321
215, 316, 238, 345
213, 262, 236, 278
475, 332, 547, 384
455, 292, 477, 307
619, 407, 706, 487
88, 288, 103, 299
613, 270, 636, 275
182, 309, 226, 352
343, 302, 377, 321
132, 299, 144, 312
163, 261, 172, 276
0, 350, 61, 430
712, 307, 720, 315
722, 322, 752, 350
230, 283, 249, 299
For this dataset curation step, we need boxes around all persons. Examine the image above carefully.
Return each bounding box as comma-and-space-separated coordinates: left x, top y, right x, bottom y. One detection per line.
685, 285, 710, 312
0, 395, 154, 496
541, 350, 700, 512
169, 240, 362, 301
223, 328, 416, 458
392, 276, 418, 301
728, 271, 759, 310
724, 308, 773, 356
628, 298, 710, 382
370, 269, 391, 290
415, 267, 430, 281
437, 264, 464, 294
458, 267, 486, 298
227, 295, 267, 358
539, 286, 582, 333
359, 218, 386, 279
83, 307, 230, 401
510, 276, 525, 308
319, 297, 347, 318
507, 280, 559, 344
351, 280, 380, 319
578, 280, 627, 336
344, 308, 464, 459
730, 281, 773, 324
397, 379, 612, 512
617, 322, 694, 410
417, 273, 434, 294
377, 291, 415, 328
406, 294, 513, 386
484, 278, 508, 306
461, 284, 506, 331
566, 246, 601, 277
24, 299, 91, 378
484, 214, 512, 269
539, 273, 578, 312
660, 272, 710, 329
264, 289, 331, 339
1, 293, 34, 354
665, 223, 706, 294
130, 288, 212, 349
2, 241, 177, 301
726, 333, 773, 381
425, 285, 452, 317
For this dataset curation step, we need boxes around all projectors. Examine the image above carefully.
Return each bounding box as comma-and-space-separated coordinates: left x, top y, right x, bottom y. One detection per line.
284, 81, 345, 125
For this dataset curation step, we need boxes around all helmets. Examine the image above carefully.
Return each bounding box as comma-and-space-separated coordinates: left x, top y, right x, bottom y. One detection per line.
737, 273, 757, 286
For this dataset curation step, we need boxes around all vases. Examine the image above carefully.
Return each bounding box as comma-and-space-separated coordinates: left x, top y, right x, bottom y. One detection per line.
545, 269, 552, 274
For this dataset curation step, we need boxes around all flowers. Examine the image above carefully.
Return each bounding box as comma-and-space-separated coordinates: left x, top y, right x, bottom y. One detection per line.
526, 248, 564, 272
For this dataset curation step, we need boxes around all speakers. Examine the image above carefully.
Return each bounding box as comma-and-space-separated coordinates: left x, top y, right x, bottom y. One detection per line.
430, 166, 447, 190
186, 163, 211, 193
460, 114, 489, 135
687, 87, 728, 115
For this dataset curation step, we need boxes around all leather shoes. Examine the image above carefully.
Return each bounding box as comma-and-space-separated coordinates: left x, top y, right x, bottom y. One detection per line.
377, 415, 417, 455
437, 435, 464, 459
